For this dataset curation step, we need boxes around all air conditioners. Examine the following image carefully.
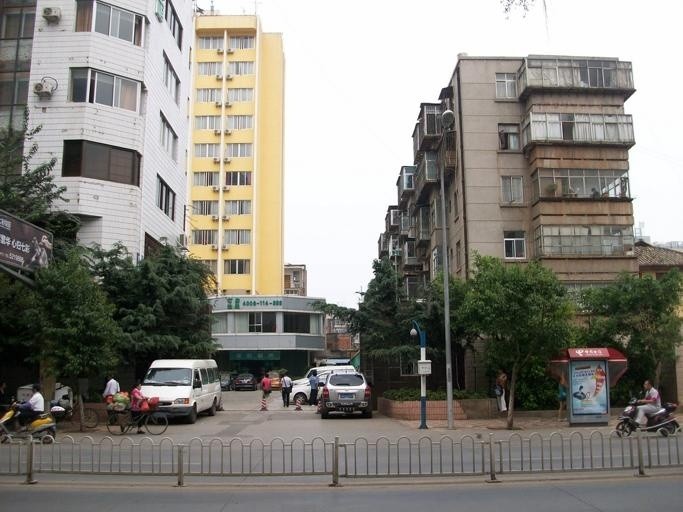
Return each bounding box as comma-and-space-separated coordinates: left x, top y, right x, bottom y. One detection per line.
212, 215, 218, 218
217, 48, 223, 53
223, 185, 229, 190
225, 101, 231, 107
224, 157, 230, 161
216, 75, 223, 79
215, 102, 221, 105
223, 244, 228, 249
227, 48, 233, 53
214, 129, 220, 133
212, 244, 217, 249
225, 129, 231, 133
213, 157, 219, 161
212, 185, 219, 190
227, 74, 232, 79
223, 215, 229, 219
42, 7, 61, 20
32, 82, 52, 95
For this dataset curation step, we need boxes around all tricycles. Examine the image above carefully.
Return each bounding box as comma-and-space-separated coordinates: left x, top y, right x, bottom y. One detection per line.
17, 382, 99, 428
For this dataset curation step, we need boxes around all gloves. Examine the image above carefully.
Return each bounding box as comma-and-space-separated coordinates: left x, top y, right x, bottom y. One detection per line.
16, 402, 31, 409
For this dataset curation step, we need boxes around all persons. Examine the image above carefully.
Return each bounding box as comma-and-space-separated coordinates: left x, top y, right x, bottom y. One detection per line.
13, 383, 44, 433
260, 373, 271, 401
305, 369, 319, 406
279, 371, 292, 407
0, 380, 6, 404
129, 380, 146, 434
101, 373, 121, 425
557, 372, 568, 422
634, 379, 662, 428
493, 370, 507, 414
573, 385, 586, 400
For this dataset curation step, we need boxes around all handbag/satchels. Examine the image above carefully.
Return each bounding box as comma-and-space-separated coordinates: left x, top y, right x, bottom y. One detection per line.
495, 385, 503, 396
286, 386, 292, 393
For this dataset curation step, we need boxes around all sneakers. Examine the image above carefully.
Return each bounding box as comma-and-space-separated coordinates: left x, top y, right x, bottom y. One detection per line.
137, 430, 145, 434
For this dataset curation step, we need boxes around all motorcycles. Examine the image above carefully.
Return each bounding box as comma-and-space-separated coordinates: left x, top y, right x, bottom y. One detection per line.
615, 390, 681, 437
0, 396, 67, 443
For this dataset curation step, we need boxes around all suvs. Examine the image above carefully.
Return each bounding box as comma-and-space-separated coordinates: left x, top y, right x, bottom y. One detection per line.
285, 365, 372, 419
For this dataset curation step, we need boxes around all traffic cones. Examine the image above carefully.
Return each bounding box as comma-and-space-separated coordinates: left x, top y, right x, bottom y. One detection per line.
260, 395, 268, 411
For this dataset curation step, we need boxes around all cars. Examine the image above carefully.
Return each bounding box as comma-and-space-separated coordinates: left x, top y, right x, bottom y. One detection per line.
221, 374, 234, 391
261, 373, 281, 390
234, 373, 257, 391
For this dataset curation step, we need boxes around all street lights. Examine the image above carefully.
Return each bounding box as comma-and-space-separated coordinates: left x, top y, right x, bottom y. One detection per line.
440, 110, 455, 429
411, 319, 429, 429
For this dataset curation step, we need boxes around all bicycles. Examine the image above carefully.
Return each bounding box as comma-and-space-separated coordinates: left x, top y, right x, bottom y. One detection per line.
106, 398, 169, 435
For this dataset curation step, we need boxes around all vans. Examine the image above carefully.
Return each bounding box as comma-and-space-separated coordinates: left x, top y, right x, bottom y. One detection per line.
139, 359, 222, 424
